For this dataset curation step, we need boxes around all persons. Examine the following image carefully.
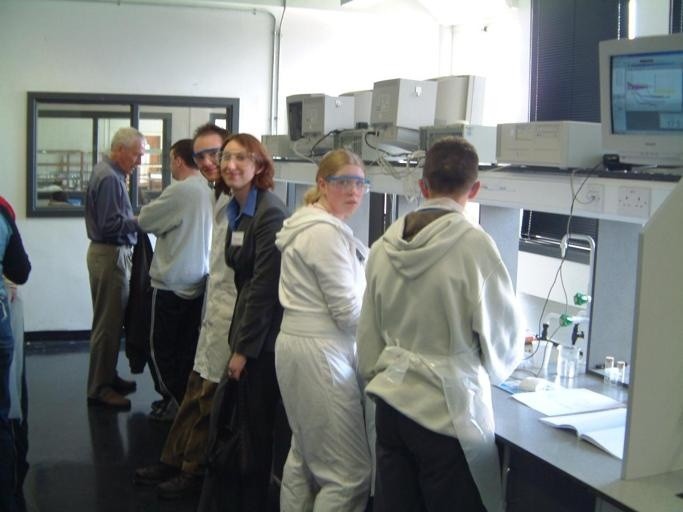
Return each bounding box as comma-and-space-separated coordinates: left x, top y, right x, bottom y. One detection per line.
275, 148, 372, 511
0, 196, 31, 512
137, 140, 213, 423
198, 135, 291, 512
138, 124, 231, 498
355, 136, 523, 512
83, 128, 146, 409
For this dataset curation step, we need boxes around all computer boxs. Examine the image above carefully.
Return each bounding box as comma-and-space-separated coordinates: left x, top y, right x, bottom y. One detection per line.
259, 134, 311, 159
418, 123, 497, 164
495, 120, 601, 171
332, 128, 412, 162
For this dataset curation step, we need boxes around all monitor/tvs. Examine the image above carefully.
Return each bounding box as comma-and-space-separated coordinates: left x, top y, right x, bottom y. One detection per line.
285, 94, 311, 143
302, 89, 373, 141
597, 33, 683, 175
368, 74, 484, 152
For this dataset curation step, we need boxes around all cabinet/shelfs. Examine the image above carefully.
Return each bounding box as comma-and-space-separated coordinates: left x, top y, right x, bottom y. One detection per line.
495, 438, 596, 512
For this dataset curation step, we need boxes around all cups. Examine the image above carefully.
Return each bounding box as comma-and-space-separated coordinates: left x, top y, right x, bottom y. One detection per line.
532, 340, 553, 368
557, 344, 582, 377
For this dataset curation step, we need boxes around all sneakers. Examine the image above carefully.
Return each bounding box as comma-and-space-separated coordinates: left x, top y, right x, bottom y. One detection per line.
151, 400, 162, 410
149, 401, 175, 421
155, 474, 200, 499
113, 376, 136, 389
134, 466, 161, 484
88, 387, 130, 406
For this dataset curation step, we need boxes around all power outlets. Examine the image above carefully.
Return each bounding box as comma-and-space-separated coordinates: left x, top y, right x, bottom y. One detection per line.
576, 182, 604, 214
616, 186, 652, 218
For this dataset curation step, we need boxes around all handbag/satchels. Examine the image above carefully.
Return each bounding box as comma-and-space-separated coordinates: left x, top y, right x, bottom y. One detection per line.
196, 368, 259, 512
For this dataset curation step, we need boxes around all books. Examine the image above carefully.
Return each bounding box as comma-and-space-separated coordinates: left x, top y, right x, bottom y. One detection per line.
509, 387, 626, 460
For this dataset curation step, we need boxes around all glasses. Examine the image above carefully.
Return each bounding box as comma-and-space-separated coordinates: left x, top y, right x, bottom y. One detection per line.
325, 175, 370, 194
192, 147, 220, 164
214, 151, 258, 166
